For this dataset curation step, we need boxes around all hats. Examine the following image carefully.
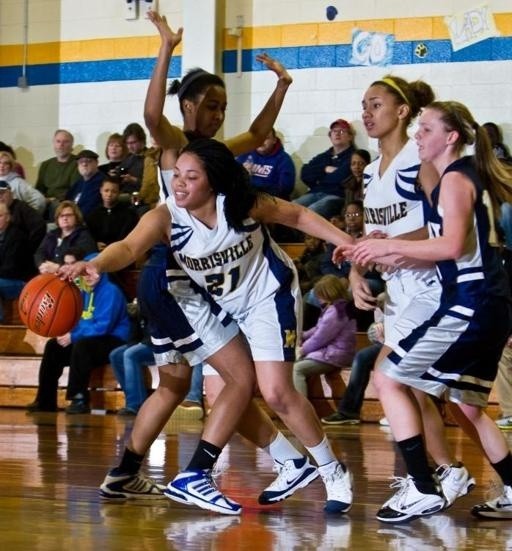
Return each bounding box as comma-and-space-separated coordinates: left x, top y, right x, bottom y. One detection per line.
76, 150, 99, 160
329, 119, 352, 132
0, 180, 12, 191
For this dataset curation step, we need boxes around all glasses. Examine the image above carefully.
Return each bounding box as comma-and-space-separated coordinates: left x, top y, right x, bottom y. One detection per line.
58, 212, 75, 217
344, 212, 363, 218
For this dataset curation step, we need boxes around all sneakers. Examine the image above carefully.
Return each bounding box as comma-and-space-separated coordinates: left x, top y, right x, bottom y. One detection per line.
98, 468, 168, 502
64, 399, 91, 415
258, 455, 319, 505
492, 417, 511, 430
379, 417, 389, 426
25, 400, 59, 412
319, 412, 361, 425
316, 461, 355, 514
116, 404, 142, 418
180, 401, 203, 408
375, 474, 447, 525
469, 486, 511, 521
163, 468, 243, 516
210, 461, 231, 480
435, 462, 476, 510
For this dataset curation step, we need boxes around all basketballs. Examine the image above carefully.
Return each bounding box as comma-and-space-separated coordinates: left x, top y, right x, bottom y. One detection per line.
18, 271, 82, 338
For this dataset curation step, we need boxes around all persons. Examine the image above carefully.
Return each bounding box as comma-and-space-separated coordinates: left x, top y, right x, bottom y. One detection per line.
330, 99, 512, 523
1, 121, 202, 419
58, 138, 387, 514
238, 120, 389, 427
96, 9, 293, 515
350, 76, 494, 508
481, 122, 511, 431
